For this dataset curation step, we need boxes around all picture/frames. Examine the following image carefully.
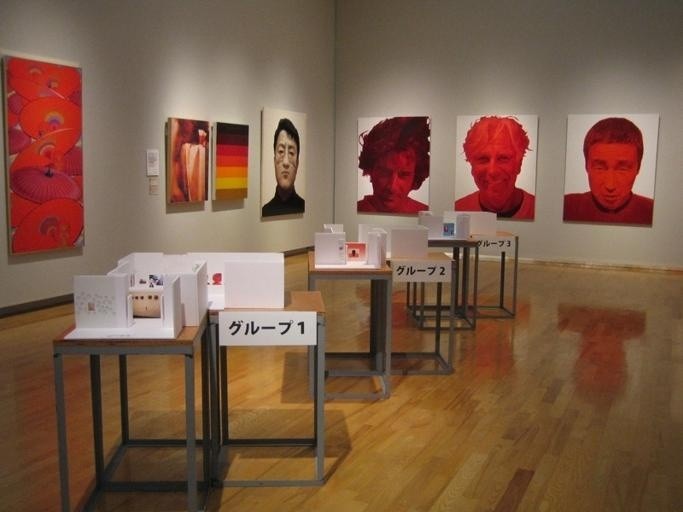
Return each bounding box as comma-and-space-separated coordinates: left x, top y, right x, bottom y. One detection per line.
1, 48, 90, 258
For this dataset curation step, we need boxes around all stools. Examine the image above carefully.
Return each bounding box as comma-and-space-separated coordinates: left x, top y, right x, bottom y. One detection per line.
401, 229, 520, 332
304, 245, 455, 400
46, 287, 326, 512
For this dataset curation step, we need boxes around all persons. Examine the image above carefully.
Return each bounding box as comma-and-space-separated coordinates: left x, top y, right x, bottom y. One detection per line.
454, 115, 535, 222
563, 118, 652, 225
356, 117, 428, 212
262, 117, 305, 214
